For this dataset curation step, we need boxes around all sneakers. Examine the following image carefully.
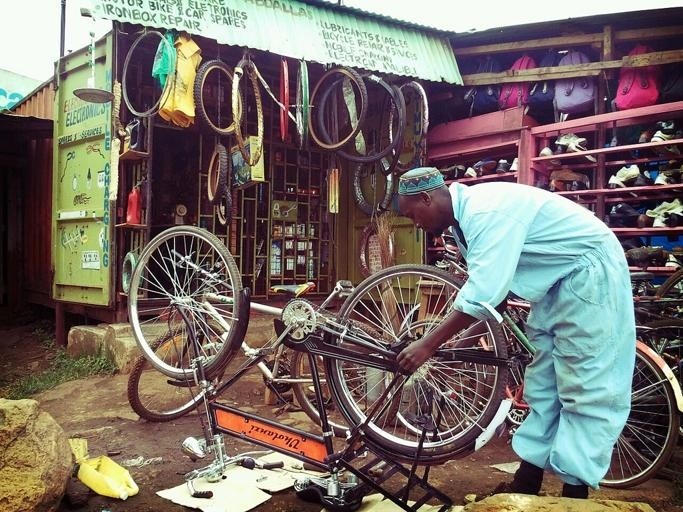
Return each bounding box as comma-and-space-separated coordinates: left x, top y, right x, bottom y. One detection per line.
440, 157, 519, 178
432, 226, 461, 268
539, 124, 682, 297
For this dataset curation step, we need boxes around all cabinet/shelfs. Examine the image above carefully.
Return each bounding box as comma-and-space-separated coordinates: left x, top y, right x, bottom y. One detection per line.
422, 140, 522, 292
529, 103, 682, 405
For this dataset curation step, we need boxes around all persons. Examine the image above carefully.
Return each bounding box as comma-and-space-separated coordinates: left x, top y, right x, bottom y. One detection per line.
397, 166, 637, 498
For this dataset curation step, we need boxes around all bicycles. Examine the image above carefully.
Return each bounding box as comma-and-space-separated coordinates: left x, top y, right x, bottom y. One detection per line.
376, 255, 683, 489
128, 224, 528, 505
131, 246, 385, 435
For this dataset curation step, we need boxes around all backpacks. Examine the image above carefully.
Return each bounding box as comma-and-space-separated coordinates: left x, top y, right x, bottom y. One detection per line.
430, 49, 683, 123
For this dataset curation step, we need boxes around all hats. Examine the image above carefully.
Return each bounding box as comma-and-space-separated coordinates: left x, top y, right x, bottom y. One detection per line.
398, 167, 446, 196
